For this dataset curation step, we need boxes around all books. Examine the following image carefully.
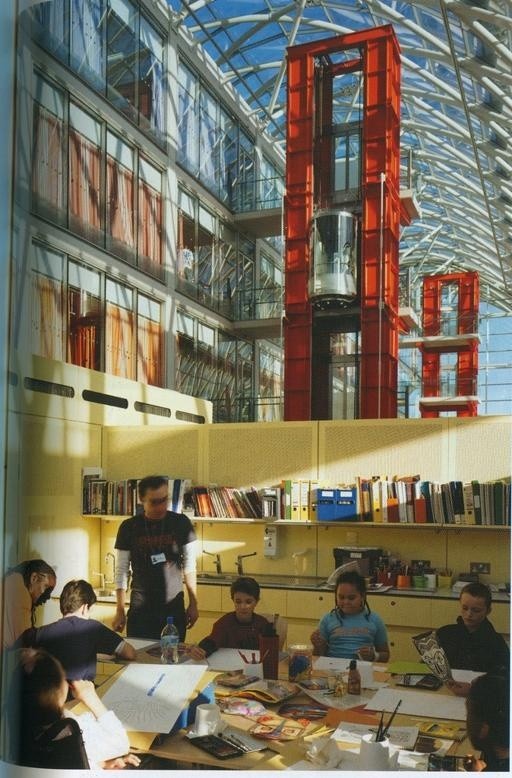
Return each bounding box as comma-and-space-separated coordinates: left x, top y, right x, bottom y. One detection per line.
82, 465, 512, 527
329, 719, 420, 750
412, 719, 469, 742
236, 678, 303, 705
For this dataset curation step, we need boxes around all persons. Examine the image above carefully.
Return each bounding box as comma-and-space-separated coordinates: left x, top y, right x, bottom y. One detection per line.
109, 473, 201, 642
1, 554, 54, 651
310, 567, 392, 663
458, 669, 508, 770
0, 645, 145, 769
420, 580, 511, 671
32, 576, 138, 702
188, 574, 274, 661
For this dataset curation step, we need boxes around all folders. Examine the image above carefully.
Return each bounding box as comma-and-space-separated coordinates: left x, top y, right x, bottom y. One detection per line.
290, 479, 320, 521
461, 481, 476, 525
370, 476, 383, 523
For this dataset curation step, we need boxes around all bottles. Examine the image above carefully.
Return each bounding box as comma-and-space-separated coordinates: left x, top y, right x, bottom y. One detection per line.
160, 616, 179, 665
348, 659, 362, 695
289, 644, 312, 681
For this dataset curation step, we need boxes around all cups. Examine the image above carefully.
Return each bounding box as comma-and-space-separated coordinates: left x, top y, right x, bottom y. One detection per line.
242, 660, 264, 683
377, 568, 452, 589
259, 634, 278, 680
195, 704, 222, 736
358, 733, 390, 771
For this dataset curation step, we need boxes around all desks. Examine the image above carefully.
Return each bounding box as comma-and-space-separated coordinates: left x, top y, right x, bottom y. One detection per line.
57, 650, 488, 770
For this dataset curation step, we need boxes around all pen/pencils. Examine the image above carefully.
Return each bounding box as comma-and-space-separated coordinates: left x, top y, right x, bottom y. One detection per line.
368, 729, 390, 738
377, 560, 435, 580
437, 567, 455, 578
238, 648, 270, 664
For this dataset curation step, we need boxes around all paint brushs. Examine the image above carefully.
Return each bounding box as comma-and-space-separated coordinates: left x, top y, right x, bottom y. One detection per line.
195, 641, 210, 666
376, 699, 402, 742
217, 732, 252, 752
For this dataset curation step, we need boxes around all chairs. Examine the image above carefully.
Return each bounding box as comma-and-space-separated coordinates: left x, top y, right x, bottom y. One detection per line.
17, 715, 99, 769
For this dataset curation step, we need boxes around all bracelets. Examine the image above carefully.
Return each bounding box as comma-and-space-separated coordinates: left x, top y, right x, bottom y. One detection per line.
372, 651, 380, 661
